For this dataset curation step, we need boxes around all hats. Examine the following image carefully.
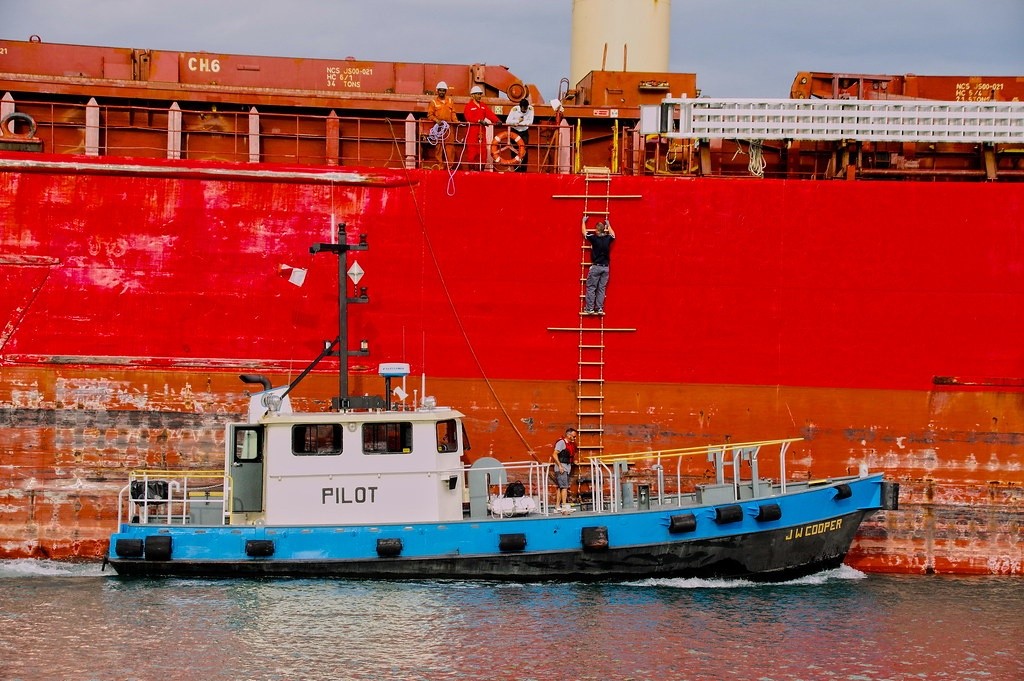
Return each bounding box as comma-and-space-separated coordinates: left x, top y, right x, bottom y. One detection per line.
470, 85, 483, 95
436, 82, 447, 89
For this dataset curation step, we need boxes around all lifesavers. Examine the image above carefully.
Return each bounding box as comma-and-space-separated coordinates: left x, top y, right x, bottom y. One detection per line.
0, 111, 38, 140
489, 131, 527, 166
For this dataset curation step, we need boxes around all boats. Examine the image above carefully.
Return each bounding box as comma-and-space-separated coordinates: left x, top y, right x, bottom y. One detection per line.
102, 222, 899, 584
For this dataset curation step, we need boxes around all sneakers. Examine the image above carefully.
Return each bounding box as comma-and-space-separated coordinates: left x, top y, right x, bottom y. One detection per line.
553, 505, 575, 515
584, 306, 594, 314
596, 307, 603, 312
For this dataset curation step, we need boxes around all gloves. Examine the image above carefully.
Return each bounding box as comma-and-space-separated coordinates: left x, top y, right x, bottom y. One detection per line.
479, 120, 489, 127
496, 120, 503, 128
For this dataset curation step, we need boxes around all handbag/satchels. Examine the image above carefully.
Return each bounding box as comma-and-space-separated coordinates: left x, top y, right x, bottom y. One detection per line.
506, 480, 525, 498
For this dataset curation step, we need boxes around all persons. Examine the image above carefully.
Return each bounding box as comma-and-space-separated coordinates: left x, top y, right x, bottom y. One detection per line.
426, 80, 461, 170
463, 86, 502, 171
505, 98, 535, 172
581, 215, 616, 314
552, 427, 578, 514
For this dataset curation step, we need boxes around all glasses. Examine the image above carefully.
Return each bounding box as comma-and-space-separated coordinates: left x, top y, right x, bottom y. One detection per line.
473, 93, 483, 95
